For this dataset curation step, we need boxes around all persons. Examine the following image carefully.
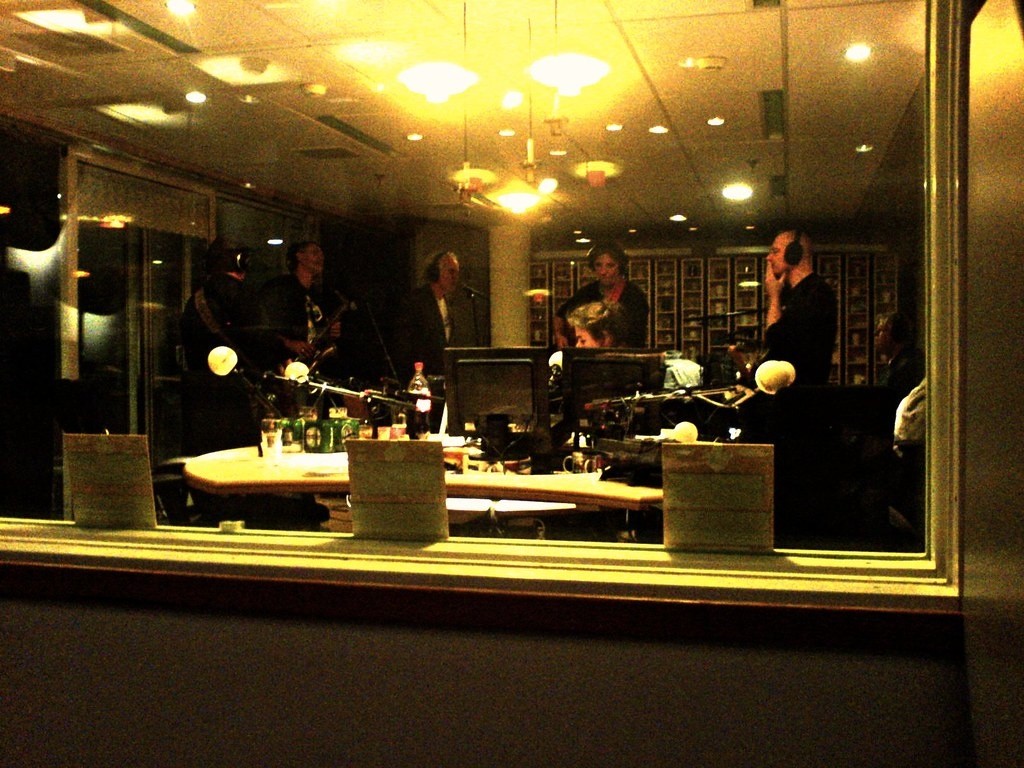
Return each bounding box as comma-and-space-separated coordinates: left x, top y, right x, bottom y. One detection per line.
764, 225, 838, 382
158, 232, 354, 525
552, 240, 649, 350
392, 249, 481, 385
873, 311, 924, 394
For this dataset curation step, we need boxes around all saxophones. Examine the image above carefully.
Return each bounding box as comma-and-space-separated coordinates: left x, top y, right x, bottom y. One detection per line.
279, 290, 349, 378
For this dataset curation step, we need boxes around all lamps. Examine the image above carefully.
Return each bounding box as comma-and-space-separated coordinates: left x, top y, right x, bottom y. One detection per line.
453, 0, 479, 215
524, 17, 536, 181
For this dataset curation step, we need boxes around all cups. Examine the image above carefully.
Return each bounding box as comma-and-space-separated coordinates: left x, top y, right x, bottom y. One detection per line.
359, 425, 373, 439
377, 427, 391, 439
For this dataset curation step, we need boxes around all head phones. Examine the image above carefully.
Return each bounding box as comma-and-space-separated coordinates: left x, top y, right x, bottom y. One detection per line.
421, 246, 447, 284
784, 227, 804, 265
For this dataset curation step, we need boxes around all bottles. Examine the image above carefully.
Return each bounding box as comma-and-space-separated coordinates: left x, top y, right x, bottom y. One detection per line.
407, 362, 431, 439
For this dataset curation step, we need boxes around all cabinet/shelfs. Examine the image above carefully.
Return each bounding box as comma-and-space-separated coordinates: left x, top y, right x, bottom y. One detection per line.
529, 258, 899, 389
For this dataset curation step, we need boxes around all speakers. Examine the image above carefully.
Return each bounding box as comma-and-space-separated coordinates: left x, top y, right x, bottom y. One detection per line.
0, 133, 61, 249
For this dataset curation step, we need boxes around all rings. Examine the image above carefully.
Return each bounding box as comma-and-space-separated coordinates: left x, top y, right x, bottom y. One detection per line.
301, 347, 305, 353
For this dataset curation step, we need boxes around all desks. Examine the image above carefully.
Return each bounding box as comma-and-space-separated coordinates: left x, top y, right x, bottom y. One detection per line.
180, 447, 351, 493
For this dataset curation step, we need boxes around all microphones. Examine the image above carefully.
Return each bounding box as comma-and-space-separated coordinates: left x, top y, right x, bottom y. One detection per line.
456, 279, 482, 295
